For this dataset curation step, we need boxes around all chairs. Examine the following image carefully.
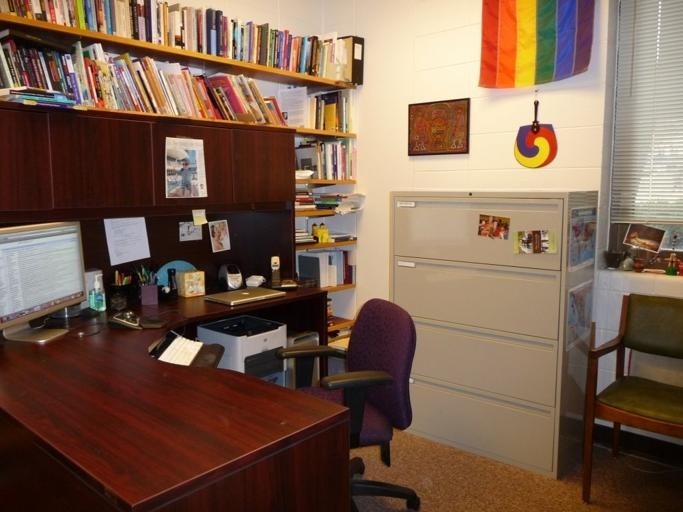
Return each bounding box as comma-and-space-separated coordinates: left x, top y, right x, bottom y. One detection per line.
291, 310, 429, 512
582, 276, 682, 506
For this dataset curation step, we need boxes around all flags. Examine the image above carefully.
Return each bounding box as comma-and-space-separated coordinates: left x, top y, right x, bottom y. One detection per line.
475, 0, 593, 90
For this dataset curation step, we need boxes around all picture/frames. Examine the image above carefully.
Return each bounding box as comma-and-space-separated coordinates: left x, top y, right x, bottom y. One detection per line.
408, 98, 471, 156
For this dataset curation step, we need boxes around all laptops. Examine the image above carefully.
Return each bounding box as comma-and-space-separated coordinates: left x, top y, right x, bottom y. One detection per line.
203, 286, 288, 307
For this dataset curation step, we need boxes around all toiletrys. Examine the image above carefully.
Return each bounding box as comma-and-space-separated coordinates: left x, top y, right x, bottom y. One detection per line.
89, 273, 108, 311
85, 269, 105, 307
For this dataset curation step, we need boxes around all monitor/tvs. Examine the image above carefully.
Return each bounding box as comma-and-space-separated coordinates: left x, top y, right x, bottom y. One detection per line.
0, 220, 90, 344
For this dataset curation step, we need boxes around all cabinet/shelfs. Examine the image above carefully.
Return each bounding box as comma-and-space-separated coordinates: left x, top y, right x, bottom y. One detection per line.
3, 0, 395, 512
388, 189, 600, 484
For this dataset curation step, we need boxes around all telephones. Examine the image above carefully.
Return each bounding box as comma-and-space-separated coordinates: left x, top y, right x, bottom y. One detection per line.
271, 256, 297, 289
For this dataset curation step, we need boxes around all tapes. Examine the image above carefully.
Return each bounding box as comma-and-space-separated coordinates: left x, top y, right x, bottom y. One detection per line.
161, 286, 170, 294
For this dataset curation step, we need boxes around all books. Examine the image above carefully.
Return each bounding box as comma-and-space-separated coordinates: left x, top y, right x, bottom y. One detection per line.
326, 297, 335, 328
1, 0, 362, 132
294, 138, 359, 289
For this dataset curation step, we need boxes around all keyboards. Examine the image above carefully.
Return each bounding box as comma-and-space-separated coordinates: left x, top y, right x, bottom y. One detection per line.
155, 334, 204, 366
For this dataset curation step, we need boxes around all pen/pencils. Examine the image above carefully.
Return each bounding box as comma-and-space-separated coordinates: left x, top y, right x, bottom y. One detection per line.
115, 270, 137, 287
136, 264, 158, 286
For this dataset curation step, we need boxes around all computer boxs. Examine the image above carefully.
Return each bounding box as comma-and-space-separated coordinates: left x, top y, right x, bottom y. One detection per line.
287, 329, 319, 388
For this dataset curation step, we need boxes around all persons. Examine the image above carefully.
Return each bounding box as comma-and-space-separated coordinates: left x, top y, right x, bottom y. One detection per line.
172, 156, 195, 198
217, 222, 228, 243
208, 225, 224, 251
477, 218, 509, 241
